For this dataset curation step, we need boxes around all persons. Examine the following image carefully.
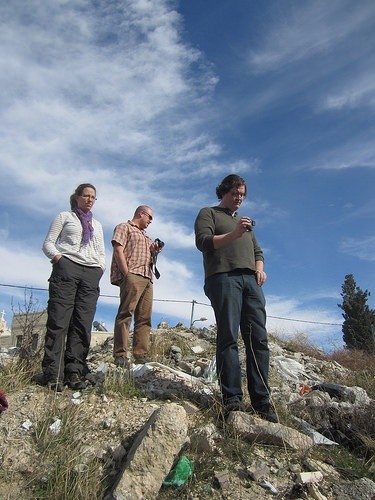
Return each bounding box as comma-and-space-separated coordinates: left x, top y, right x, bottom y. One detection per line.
112, 205, 161, 369
42, 184, 105, 392
194, 175, 278, 423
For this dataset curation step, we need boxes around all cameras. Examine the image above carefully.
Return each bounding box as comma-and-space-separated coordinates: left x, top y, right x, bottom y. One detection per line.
246, 221, 255, 231
154, 239, 164, 248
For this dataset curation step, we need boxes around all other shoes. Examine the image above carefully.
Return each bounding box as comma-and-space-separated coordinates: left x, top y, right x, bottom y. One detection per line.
253, 402, 278, 423
222, 400, 246, 414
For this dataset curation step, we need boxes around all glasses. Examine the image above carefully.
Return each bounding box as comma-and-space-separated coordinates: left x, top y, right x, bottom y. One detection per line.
143, 211, 153, 221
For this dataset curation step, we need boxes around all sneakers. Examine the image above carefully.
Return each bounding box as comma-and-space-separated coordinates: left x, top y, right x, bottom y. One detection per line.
114, 357, 130, 369
68, 374, 86, 389
47, 378, 64, 392
134, 359, 147, 364
